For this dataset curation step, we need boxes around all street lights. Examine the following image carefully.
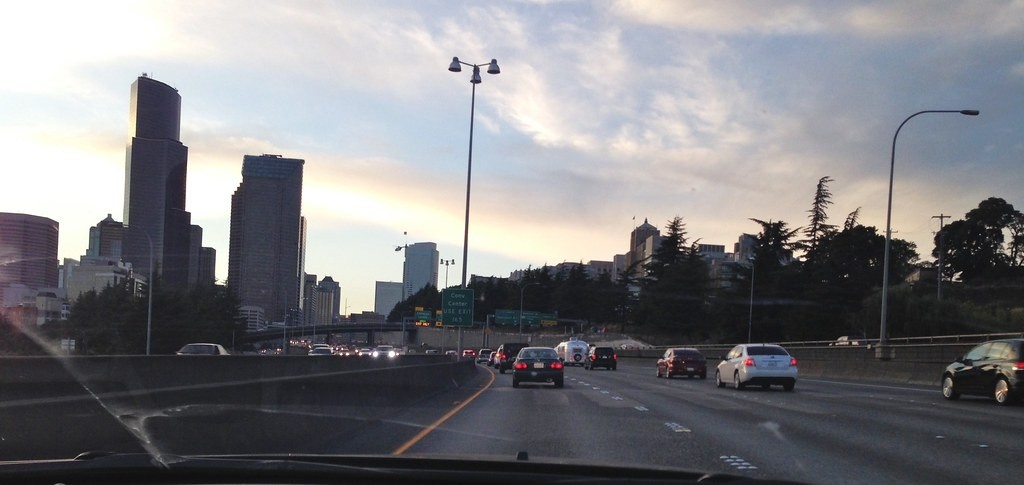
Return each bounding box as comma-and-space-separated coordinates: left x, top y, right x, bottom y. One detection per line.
881, 108, 980, 341
721, 260, 754, 344
439, 258, 455, 354
396, 243, 414, 321
448, 57, 500, 362
106, 222, 153, 356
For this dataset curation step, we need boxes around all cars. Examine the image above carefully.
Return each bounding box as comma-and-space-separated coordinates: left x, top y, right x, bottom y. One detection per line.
307, 343, 456, 360
487, 352, 496, 366
828, 336, 861, 347
941, 339, 1023, 405
175, 343, 232, 356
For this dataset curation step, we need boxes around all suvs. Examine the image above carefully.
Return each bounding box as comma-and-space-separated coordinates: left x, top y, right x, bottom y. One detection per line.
462, 349, 475, 360
478, 349, 492, 363
655, 348, 708, 379
584, 346, 617, 371
715, 342, 798, 391
493, 342, 530, 374
510, 347, 565, 388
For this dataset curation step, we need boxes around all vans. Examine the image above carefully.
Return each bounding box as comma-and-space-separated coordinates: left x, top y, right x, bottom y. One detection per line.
553, 338, 590, 366
